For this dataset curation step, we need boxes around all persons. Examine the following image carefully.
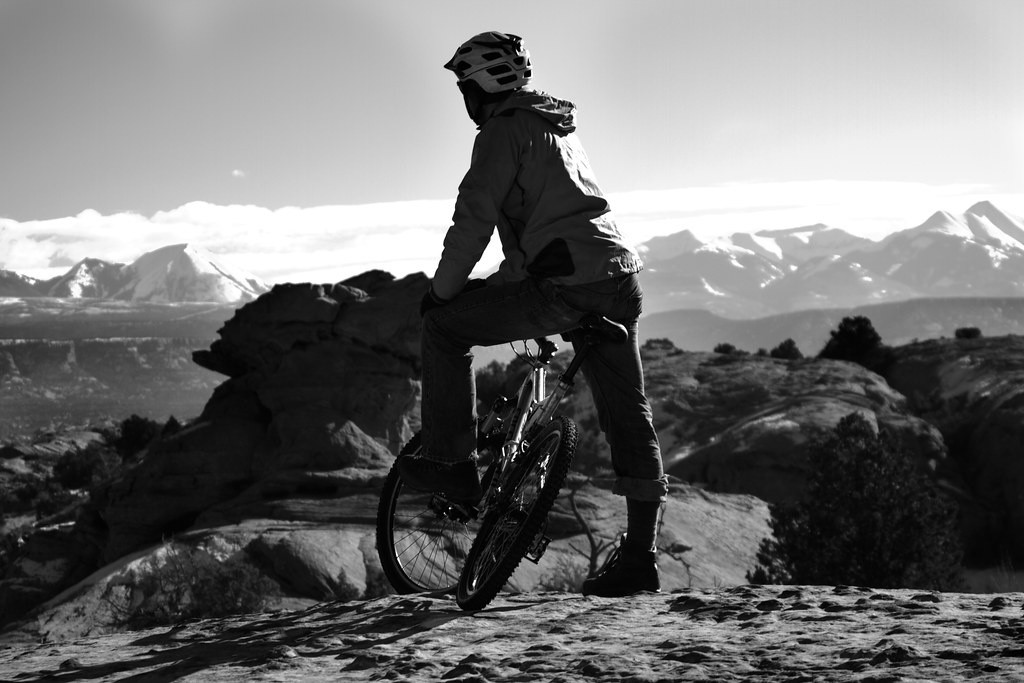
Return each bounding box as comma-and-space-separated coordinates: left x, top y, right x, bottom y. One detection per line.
418, 31, 668, 598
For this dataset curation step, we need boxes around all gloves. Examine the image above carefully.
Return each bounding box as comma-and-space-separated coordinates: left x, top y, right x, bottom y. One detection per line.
420, 293, 434, 319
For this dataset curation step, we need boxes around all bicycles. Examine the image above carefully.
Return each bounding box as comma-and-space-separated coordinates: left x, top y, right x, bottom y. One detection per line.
375, 313, 632, 614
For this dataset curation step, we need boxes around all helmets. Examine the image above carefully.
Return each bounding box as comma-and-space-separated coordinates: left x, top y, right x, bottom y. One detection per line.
444, 31, 534, 93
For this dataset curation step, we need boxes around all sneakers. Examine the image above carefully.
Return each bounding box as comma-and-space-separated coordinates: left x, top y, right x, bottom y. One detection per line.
582, 535, 661, 597
399, 455, 481, 506
